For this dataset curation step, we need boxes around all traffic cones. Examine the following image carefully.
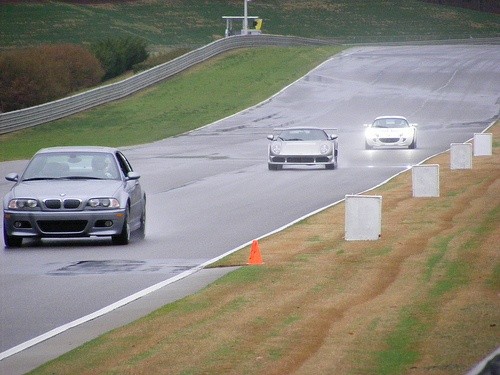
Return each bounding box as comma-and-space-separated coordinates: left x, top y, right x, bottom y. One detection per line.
246, 240, 264, 266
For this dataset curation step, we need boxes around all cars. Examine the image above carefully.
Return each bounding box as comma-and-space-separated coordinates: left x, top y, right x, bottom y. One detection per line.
465, 348, 500, 375
266, 126, 339, 172
2, 145, 146, 249
363, 116, 418, 150
